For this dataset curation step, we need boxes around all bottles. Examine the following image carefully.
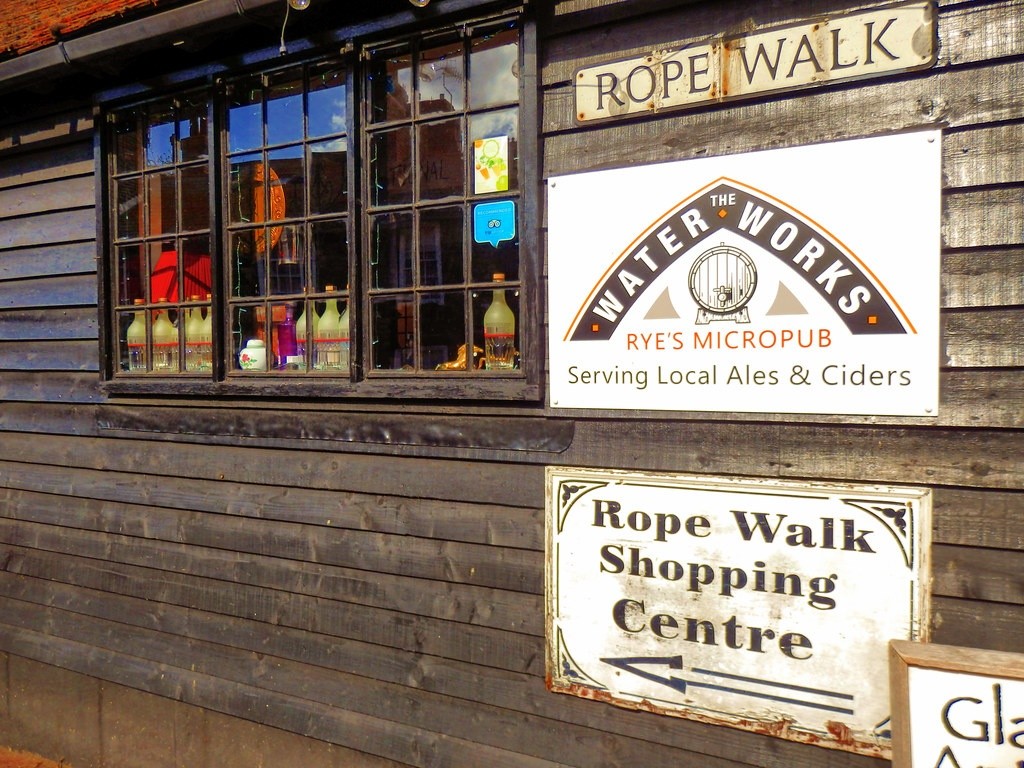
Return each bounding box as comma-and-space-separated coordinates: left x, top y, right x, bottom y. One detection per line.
483, 273, 516, 369
239, 339, 274, 373
277, 283, 350, 373
125, 293, 216, 374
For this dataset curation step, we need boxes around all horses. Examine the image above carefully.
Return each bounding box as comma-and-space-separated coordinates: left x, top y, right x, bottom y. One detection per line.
436, 345, 486, 371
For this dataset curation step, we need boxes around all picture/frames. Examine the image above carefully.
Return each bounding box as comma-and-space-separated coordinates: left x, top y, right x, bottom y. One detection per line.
887, 639, 1024, 768
